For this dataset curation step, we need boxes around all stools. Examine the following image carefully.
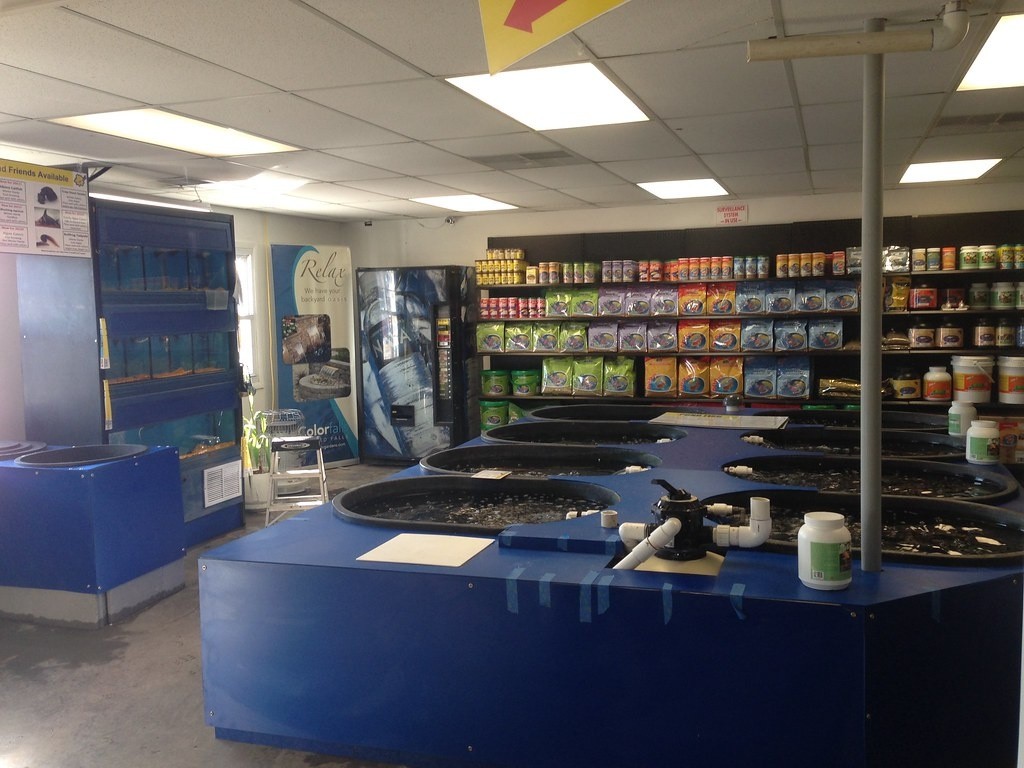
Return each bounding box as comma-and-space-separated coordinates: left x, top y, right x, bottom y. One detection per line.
265, 436, 329, 529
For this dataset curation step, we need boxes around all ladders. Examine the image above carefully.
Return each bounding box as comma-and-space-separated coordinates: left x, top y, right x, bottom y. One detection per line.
264, 437, 329, 528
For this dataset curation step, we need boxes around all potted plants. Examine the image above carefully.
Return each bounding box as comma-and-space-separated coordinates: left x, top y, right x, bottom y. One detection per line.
239, 394, 272, 511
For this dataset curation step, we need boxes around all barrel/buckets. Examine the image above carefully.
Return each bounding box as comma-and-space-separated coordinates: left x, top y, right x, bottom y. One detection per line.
951, 355, 995, 403
998, 356, 1024, 404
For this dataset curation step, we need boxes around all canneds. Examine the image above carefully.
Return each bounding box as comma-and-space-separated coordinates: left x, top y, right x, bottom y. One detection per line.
776, 251, 846, 278
912, 244, 1024, 270
474, 247, 769, 280
479, 296, 546, 319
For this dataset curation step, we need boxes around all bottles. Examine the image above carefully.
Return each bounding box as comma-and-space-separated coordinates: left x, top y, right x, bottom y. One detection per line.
362, 283, 450, 457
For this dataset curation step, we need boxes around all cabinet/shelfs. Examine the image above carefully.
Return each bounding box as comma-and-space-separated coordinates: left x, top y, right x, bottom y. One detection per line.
15, 197, 246, 549
476, 209, 1023, 405
